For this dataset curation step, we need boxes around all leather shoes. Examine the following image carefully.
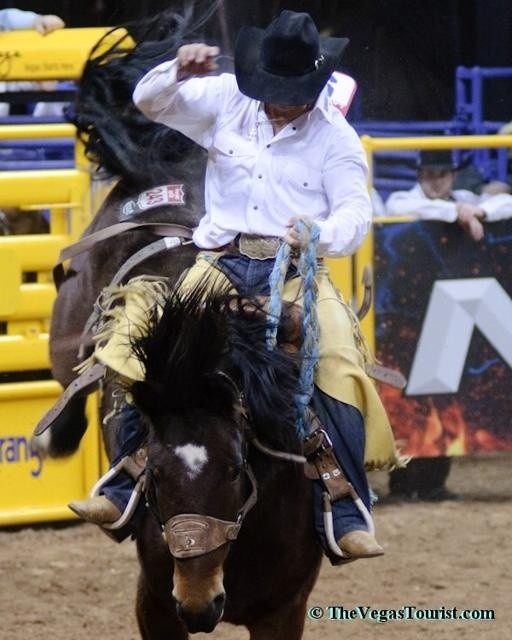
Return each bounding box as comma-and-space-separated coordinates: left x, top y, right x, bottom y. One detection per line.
68, 496, 134, 543
375, 491, 414, 505
419, 486, 456, 502
328, 531, 383, 565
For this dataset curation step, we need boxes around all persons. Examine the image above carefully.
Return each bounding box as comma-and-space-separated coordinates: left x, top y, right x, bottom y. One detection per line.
2, 10, 511, 507
70, 6, 387, 567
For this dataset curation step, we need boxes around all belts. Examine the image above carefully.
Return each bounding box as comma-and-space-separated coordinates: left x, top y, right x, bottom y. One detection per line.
209, 233, 325, 263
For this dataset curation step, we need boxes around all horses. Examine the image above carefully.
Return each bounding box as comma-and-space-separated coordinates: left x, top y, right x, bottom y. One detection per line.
26, 1, 326, 640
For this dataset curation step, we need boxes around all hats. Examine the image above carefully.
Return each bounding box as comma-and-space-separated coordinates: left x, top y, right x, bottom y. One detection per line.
234, 9, 350, 104
407, 149, 473, 171
